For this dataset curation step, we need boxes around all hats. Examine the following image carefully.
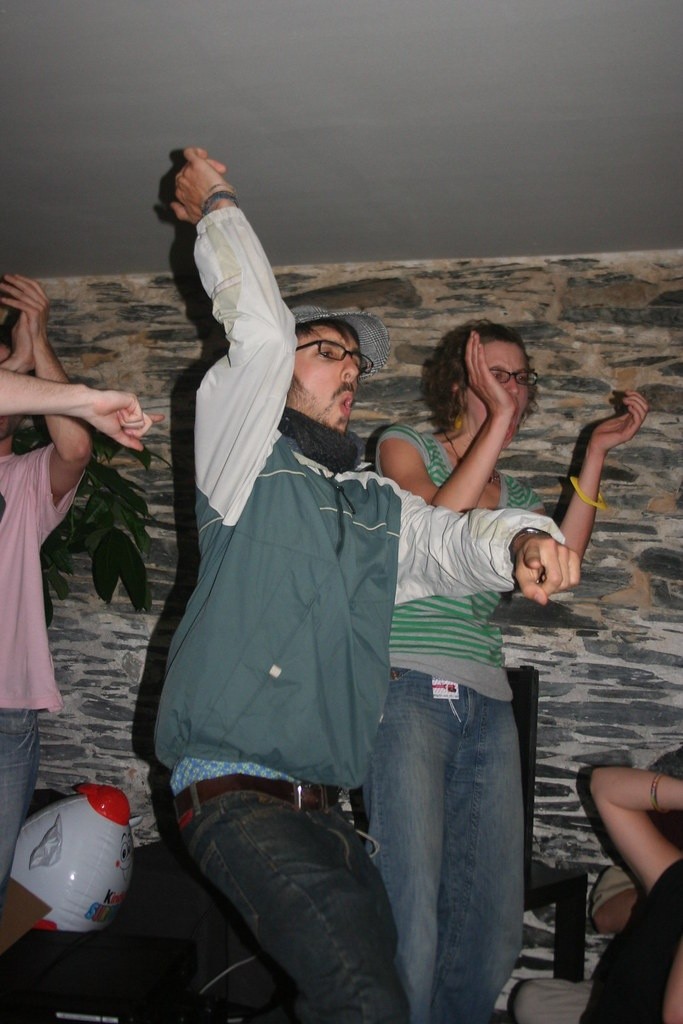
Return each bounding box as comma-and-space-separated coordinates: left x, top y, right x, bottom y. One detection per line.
290, 304, 390, 381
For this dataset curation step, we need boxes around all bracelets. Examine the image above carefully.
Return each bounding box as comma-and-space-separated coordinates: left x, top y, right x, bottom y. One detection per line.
650, 772, 667, 814
509, 527, 552, 552
570, 476, 607, 511
201, 183, 241, 217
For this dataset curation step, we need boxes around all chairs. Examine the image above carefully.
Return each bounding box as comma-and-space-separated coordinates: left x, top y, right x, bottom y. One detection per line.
323, 663, 585, 985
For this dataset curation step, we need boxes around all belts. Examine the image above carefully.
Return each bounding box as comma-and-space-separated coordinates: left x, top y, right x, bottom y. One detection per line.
173, 774, 339, 821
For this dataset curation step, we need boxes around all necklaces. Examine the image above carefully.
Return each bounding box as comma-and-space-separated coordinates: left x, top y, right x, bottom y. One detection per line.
443, 431, 499, 483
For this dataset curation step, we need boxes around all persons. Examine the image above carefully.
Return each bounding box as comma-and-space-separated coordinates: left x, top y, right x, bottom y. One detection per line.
153, 146, 578, 1024
360, 319, 683, 1024
0, 275, 164, 896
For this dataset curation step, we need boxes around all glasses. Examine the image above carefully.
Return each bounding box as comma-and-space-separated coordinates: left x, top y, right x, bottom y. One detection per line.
489, 368, 538, 386
292, 339, 373, 377
